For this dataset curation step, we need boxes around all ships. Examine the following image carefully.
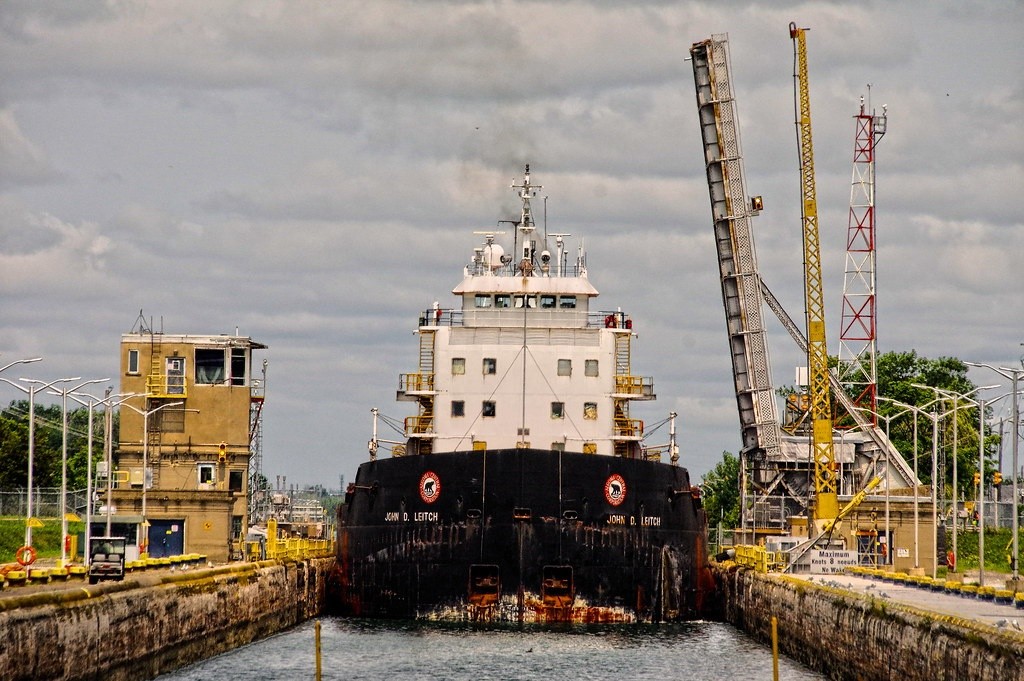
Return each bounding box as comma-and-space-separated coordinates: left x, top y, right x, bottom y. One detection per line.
323, 160, 713, 631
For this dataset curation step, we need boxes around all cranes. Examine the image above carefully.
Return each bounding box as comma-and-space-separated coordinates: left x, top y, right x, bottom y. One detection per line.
788, 24, 847, 556
689, 34, 922, 521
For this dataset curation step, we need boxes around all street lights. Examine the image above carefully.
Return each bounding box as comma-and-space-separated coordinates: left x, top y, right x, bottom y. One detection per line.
910, 380, 1024, 584
0, 375, 82, 571
848, 405, 915, 567
24, 376, 111, 567
112, 398, 185, 546
892, 400, 979, 582
830, 424, 874, 495
873, 393, 953, 568
70, 390, 157, 532
963, 360, 1024, 581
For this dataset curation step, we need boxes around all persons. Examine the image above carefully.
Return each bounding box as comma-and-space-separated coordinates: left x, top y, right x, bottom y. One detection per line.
974, 511, 980, 525
94, 541, 108, 554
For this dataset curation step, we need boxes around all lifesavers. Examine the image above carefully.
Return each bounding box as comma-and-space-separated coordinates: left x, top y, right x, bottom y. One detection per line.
604, 316, 618, 329
16, 546, 36, 566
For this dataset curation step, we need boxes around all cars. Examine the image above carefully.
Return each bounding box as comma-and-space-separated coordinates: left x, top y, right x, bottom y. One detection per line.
88, 536, 126, 586
